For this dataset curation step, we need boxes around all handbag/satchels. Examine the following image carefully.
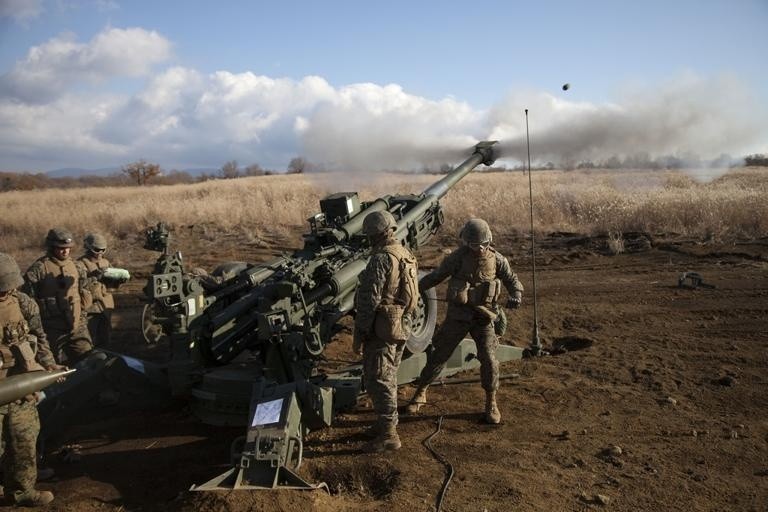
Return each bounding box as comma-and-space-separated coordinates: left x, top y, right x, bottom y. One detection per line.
373, 303, 408, 342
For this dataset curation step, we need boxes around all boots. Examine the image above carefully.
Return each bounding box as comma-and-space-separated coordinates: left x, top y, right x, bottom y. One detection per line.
406, 388, 428, 414
12, 488, 55, 508
361, 430, 402, 453
484, 390, 501, 424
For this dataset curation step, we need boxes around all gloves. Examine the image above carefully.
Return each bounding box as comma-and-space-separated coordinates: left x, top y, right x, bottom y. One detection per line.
351, 324, 366, 356
505, 295, 521, 310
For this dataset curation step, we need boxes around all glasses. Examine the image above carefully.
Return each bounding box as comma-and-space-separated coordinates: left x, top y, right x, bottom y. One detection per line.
91, 247, 107, 254
463, 240, 491, 252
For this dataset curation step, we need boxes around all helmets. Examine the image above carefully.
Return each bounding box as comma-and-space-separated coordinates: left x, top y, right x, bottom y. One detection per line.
85, 232, 108, 249
46, 228, 75, 248
361, 211, 397, 235
458, 218, 493, 243
0, 253, 26, 292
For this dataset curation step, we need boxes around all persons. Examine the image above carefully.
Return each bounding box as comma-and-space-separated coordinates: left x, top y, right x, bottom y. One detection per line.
0, 252, 71, 507
405, 217, 525, 425
17, 226, 94, 363
350, 210, 420, 454
74, 231, 127, 352
